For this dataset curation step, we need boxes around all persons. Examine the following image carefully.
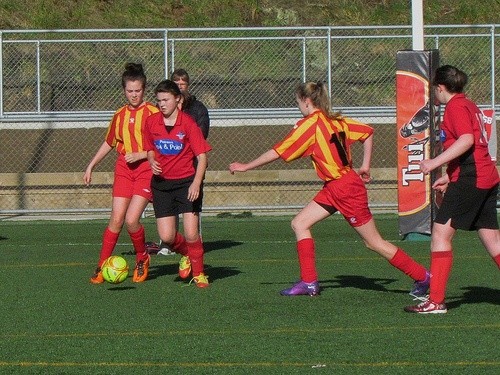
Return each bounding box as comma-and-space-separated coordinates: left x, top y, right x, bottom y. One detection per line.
229, 80, 430, 298
144, 80, 212, 289
155, 69, 210, 256
83, 62, 160, 284
402, 65, 500, 314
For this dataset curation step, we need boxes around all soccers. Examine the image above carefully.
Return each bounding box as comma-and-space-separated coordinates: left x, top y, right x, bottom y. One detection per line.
101, 255, 129, 284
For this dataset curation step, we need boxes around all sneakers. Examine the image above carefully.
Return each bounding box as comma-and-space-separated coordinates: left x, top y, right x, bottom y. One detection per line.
179, 256, 191, 278
404, 298, 447, 314
280, 280, 320, 296
90, 266, 104, 284
133, 254, 151, 282
410, 269, 430, 296
192, 272, 209, 288
